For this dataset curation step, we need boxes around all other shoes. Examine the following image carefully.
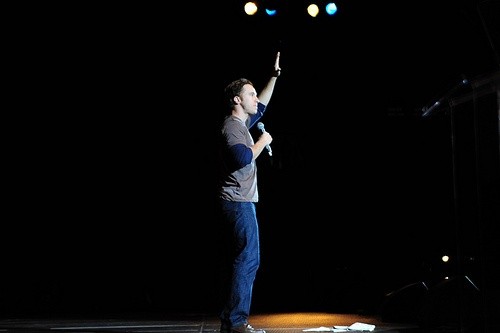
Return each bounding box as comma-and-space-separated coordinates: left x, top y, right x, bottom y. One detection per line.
220, 324, 266, 333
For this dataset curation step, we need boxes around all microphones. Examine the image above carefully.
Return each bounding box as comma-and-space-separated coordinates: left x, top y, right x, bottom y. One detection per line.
257, 122, 273, 157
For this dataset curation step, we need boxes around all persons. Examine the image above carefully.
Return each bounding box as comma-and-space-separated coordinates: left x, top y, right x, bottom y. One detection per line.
213, 51, 281, 333
